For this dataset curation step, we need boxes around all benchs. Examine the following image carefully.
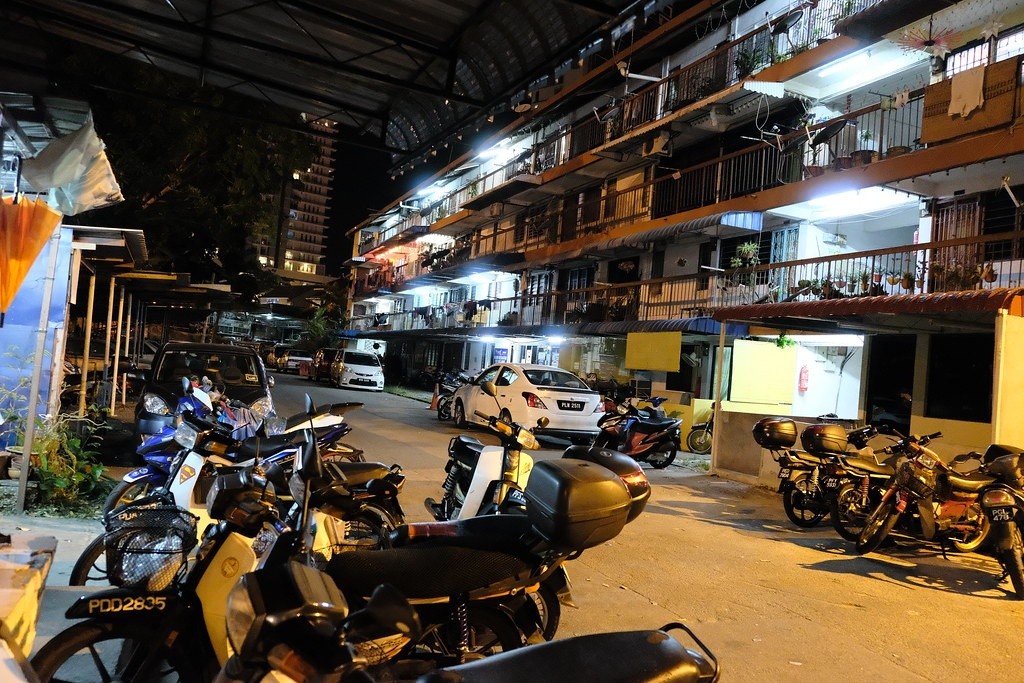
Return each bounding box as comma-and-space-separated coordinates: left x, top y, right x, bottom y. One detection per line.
530, 378, 581, 388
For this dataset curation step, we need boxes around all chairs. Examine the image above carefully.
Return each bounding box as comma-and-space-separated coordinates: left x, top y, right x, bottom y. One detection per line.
170, 353, 193, 376
219, 354, 242, 379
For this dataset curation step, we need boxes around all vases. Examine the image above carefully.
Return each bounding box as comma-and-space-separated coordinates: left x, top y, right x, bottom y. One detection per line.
6, 446, 56, 468
832, 157, 851, 172
791, 287, 799, 294
887, 146, 912, 158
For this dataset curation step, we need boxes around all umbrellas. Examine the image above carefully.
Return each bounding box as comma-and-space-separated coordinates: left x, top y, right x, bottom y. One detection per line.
0, 154, 64, 328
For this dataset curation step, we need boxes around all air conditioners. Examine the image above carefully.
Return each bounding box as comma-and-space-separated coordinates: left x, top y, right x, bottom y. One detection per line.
488, 203, 503, 217
642, 131, 670, 157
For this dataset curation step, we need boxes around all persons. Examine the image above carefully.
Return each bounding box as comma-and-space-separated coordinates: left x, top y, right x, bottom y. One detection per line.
280, 350, 289, 374
311, 350, 324, 381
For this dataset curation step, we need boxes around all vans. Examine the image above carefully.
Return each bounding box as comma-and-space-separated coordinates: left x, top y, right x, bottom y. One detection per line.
133, 340, 275, 468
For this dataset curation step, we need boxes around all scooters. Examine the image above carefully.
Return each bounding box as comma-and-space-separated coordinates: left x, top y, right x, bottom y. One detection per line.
686, 400, 716, 455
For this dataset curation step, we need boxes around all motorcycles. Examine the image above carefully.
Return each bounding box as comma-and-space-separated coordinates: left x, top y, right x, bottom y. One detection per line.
856, 422, 999, 562
965, 448, 1024, 600
589, 389, 684, 470
800, 421, 944, 544
602, 382, 669, 448
752, 415, 880, 530
26, 376, 723, 683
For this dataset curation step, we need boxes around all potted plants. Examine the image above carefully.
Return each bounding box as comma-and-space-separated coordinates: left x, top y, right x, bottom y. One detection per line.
813, 28, 832, 45
798, 262, 998, 295
851, 130, 877, 167
802, 146, 824, 179
736, 240, 762, 258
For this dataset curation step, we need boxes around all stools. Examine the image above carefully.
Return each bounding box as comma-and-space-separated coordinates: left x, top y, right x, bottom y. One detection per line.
680, 305, 704, 318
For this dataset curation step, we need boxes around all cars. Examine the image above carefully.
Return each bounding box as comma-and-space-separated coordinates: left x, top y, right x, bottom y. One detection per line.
329, 348, 386, 393
307, 347, 339, 383
452, 363, 607, 447
275, 348, 314, 375
65, 323, 293, 374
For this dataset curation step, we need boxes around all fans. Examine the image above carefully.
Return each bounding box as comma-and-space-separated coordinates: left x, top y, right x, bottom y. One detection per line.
682, 352, 702, 368
